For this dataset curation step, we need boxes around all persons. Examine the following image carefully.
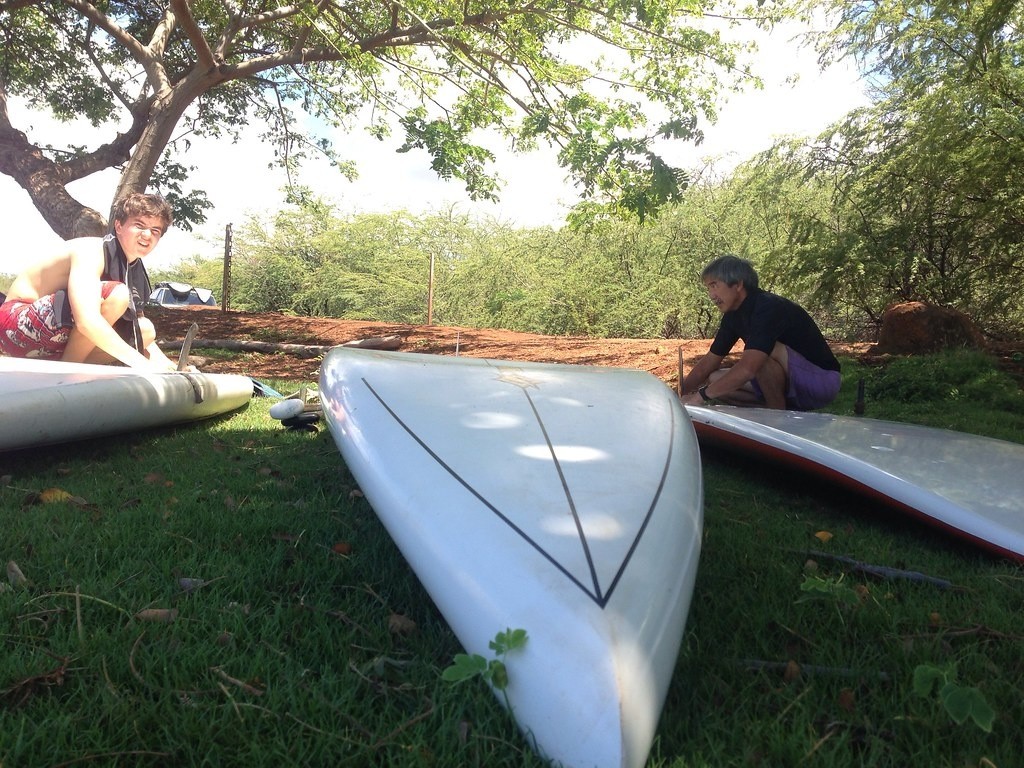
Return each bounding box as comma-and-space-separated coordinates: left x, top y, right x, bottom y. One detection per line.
674, 255, 841, 411
0, 193, 202, 374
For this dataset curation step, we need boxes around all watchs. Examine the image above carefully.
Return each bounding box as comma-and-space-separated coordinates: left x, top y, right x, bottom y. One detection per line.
698, 384, 712, 402
